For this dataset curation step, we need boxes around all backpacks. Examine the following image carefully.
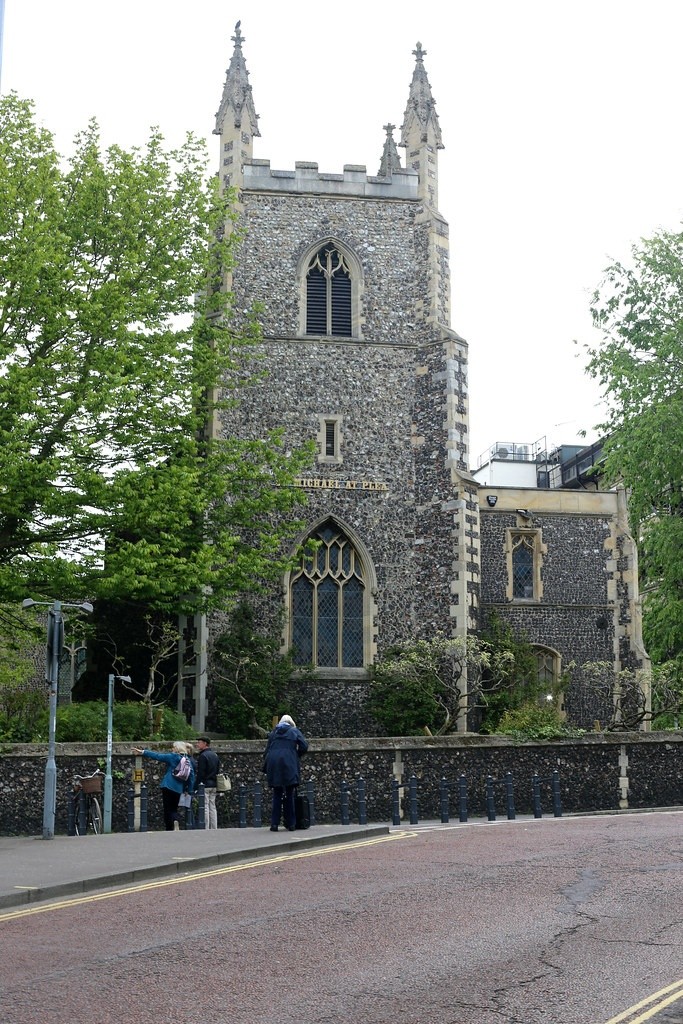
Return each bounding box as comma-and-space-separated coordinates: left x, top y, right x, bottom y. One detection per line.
171, 754, 190, 781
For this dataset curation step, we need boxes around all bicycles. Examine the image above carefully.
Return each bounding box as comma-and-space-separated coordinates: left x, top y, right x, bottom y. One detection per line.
68, 769, 103, 835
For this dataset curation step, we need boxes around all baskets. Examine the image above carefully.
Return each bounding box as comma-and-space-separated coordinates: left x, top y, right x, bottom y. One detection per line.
81, 776, 102, 793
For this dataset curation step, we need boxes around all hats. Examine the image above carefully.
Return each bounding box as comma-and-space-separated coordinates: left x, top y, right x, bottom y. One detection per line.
194, 737, 210, 744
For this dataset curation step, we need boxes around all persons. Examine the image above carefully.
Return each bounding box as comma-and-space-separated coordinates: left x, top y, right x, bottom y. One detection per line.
131, 736, 220, 831
263, 714, 308, 831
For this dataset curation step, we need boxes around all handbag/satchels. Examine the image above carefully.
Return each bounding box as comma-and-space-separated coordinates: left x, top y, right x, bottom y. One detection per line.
282, 785, 310, 829
216, 773, 230, 791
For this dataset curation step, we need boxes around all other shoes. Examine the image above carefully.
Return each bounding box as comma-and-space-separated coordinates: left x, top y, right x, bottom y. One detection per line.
269, 828, 278, 831
173, 820, 180, 831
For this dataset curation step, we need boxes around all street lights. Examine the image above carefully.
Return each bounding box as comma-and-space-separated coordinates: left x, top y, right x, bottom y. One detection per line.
21, 597, 94, 841
103, 674, 132, 834
